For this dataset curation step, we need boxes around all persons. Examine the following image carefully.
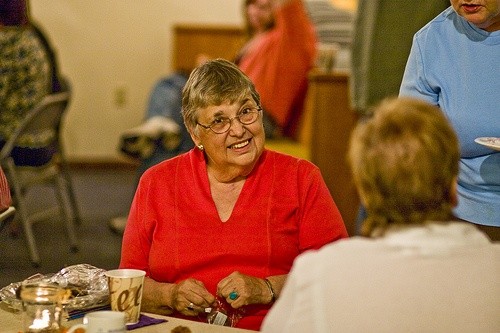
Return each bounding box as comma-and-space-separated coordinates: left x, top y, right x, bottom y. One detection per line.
257, 94, 500, 333
109, 0, 317, 236
0, 0, 61, 238
118, 55, 350, 333
394, 0, 500, 242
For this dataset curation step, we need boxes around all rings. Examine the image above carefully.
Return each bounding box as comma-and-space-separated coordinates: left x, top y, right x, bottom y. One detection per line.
229, 291, 238, 300
188, 302, 194, 310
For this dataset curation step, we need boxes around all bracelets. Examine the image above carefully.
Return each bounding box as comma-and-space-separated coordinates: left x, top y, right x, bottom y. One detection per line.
262, 277, 274, 301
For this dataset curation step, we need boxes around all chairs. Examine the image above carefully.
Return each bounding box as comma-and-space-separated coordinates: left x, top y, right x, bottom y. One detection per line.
0, 77, 81, 266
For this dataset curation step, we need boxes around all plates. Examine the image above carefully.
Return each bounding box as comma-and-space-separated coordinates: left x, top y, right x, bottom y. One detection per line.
474, 136, 500, 152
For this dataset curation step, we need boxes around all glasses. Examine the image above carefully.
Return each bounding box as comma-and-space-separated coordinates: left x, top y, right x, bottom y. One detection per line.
196, 108, 263, 133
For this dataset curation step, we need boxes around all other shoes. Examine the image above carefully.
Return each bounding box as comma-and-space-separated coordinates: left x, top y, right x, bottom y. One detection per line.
109, 217, 128, 234
119, 117, 185, 161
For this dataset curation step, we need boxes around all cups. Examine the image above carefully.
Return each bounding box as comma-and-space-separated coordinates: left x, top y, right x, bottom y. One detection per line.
106, 268, 147, 325
16, 277, 71, 333
65, 310, 127, 333
